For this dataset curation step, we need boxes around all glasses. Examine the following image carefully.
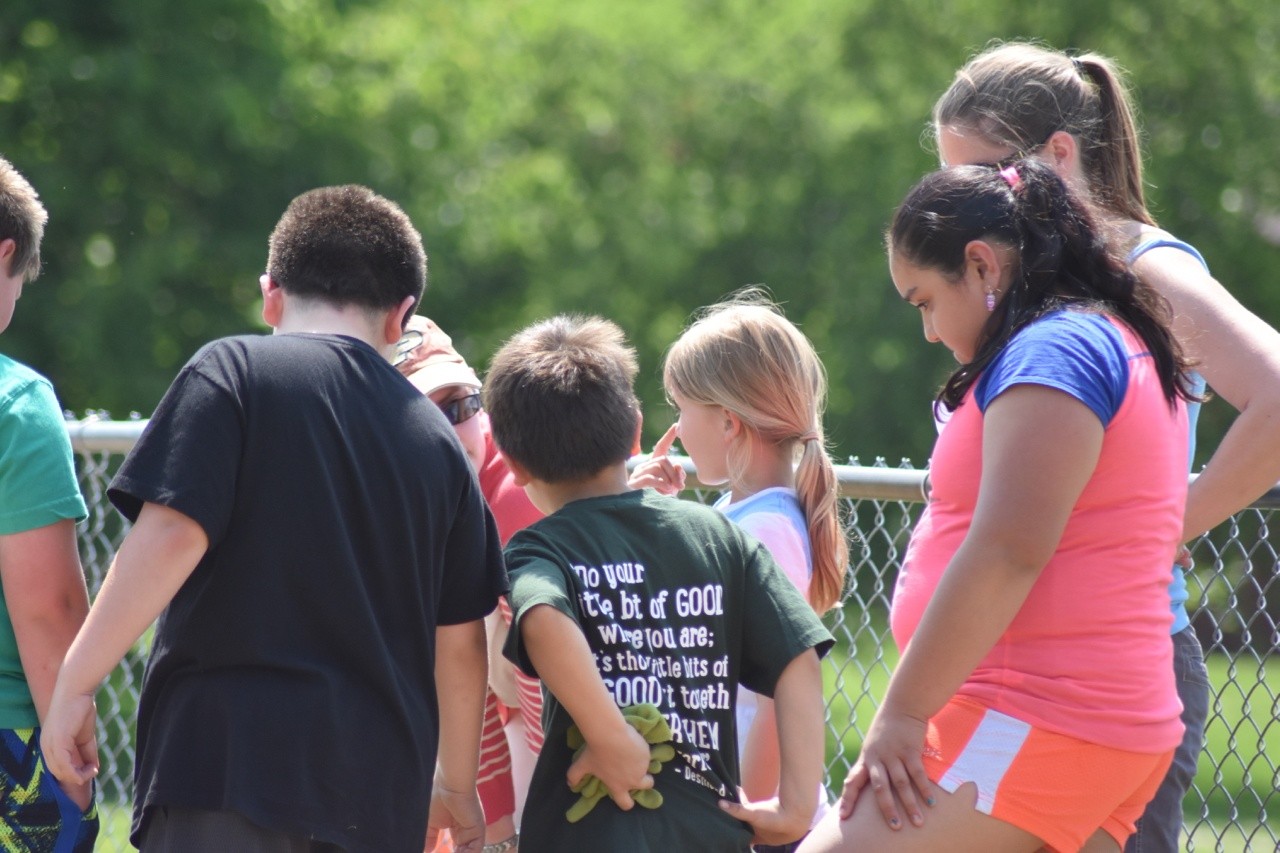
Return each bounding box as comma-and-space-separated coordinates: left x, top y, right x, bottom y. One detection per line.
434, 386, 482, 425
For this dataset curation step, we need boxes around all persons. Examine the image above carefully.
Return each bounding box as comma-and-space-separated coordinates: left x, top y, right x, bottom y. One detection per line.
379, 317, 553, 853
930, 39, 1280, 853
623, 284, 847, 853
484, 314, 837, 853
0, 153, 104, 853
791, 159, 1186, 853
38, 182, 511, 853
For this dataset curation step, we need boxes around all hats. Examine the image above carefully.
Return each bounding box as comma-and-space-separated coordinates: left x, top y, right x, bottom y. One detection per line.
382, 313, 483, 396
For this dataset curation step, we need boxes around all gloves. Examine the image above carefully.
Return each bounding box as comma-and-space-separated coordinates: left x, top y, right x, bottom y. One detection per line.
566, 703, 675, 823
